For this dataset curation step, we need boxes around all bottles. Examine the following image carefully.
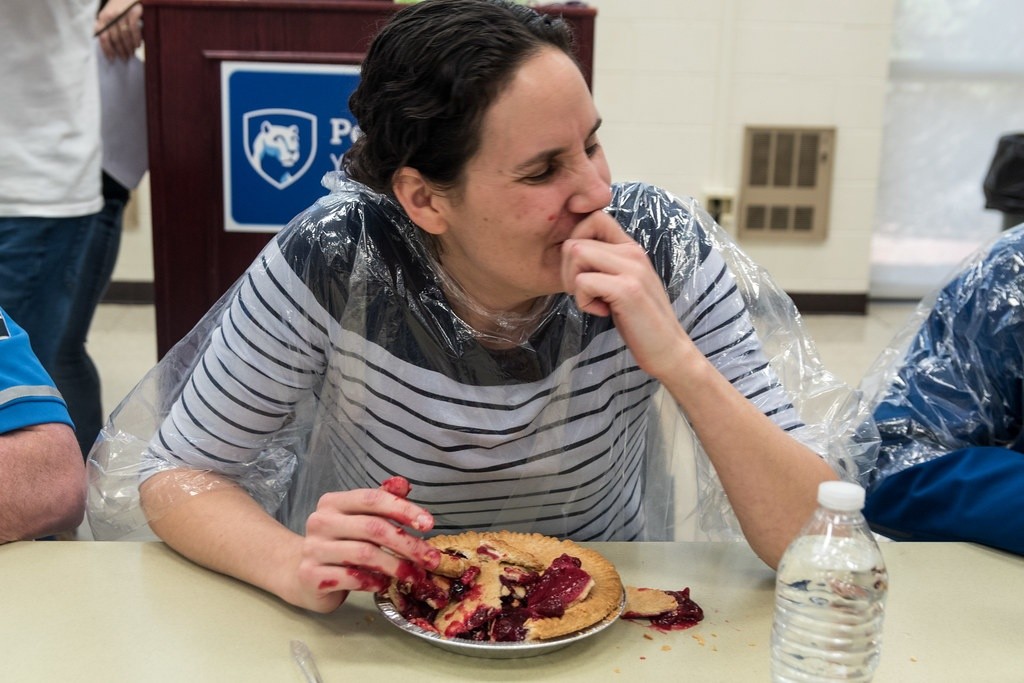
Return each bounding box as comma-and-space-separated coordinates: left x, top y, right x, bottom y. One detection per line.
770, 480, 889, 683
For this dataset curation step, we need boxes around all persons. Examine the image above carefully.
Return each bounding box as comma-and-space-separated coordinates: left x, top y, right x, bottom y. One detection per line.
1, 308, 87, 546
843, 222, 1024, 558
0, 1, 104, 380
140, 0, 849, 620
62, 0, 148, 469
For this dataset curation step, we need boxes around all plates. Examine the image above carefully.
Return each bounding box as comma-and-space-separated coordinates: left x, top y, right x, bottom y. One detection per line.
374, 584, 626, 659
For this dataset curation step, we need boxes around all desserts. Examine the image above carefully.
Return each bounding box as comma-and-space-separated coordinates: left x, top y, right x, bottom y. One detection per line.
372, 531, 705, 659
